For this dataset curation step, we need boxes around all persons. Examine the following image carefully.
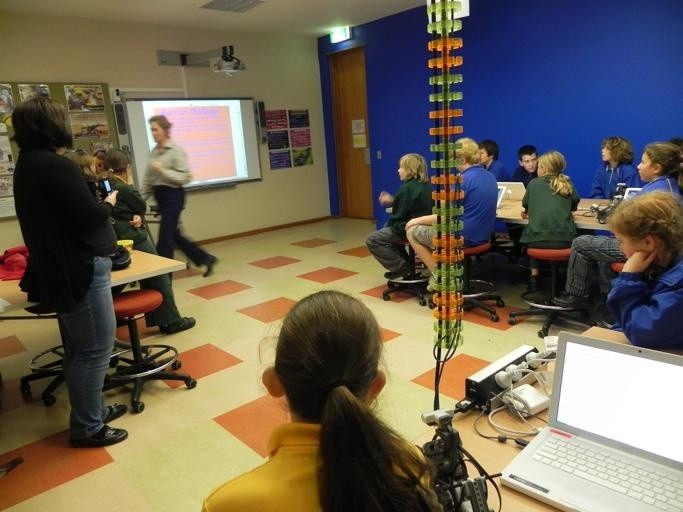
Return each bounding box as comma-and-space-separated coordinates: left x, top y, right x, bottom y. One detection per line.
519, 150, 580, 293
365, 152, 436, 279
478, 140, 511, 233
587, 135, 640, 199
71, 154, 98, 177
140, 114, 218, 278
102, 147, 196, 335
403, 137, 498, 293
198, 287, 447, 512
10, 96, 130, 448
511, 144, 539, 190
549, 140, 683, 309
607, 190, 683, 350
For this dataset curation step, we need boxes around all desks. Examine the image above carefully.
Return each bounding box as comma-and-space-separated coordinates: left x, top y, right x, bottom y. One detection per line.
412, 326, 683, 512
0, 249, 187, 354
386, 198, 617, 230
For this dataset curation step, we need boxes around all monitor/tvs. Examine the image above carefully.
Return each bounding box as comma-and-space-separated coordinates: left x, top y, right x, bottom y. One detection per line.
495, 186, 506, 209
623, 187, 642, 200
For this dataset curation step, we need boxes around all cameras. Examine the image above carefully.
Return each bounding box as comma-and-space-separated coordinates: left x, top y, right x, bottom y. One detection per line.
98, 177, 112, 201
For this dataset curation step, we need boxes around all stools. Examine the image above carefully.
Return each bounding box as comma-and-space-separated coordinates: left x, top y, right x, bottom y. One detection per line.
428, 243, 505, 322
102, 290, 197, 413
383, 240, 429, 306
508, 248, 597, 338
590, 262, 625, 329
21, 292, 115, 406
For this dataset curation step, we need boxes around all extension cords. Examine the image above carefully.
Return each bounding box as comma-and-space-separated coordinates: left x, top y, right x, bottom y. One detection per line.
463, 344, 539, 414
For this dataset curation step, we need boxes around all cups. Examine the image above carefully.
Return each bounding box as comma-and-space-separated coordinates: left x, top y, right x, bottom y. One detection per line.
116, 240, 134, 255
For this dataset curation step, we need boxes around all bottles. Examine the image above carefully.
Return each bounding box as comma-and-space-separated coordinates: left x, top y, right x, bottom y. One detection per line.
98, 175, 114, 200
614, 183, 626, 201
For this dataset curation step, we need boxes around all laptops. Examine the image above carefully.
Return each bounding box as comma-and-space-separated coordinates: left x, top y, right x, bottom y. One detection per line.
499, 331, 682, 512
496, 182, 525, 200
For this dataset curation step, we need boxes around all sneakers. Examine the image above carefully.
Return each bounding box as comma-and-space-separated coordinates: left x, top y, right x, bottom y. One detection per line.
385, 265, 408, 279
552, 293, 589, 306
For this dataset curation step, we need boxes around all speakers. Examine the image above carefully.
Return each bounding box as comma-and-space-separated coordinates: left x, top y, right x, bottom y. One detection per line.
254, 100, 268, 145
112, 102, 130, 152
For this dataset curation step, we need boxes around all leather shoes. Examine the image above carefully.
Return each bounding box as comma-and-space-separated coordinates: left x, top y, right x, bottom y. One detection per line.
70, 425, 128, 447
203, 257, 217, 277
103, 403, 127, 423
166, 317, 195, 333
526, 275, 538, 293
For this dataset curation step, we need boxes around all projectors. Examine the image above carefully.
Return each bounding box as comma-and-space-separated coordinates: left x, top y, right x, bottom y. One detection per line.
210, 55, 246, 74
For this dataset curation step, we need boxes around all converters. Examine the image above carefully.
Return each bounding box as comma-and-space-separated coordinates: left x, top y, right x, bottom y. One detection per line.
503, 383, 549, 417
